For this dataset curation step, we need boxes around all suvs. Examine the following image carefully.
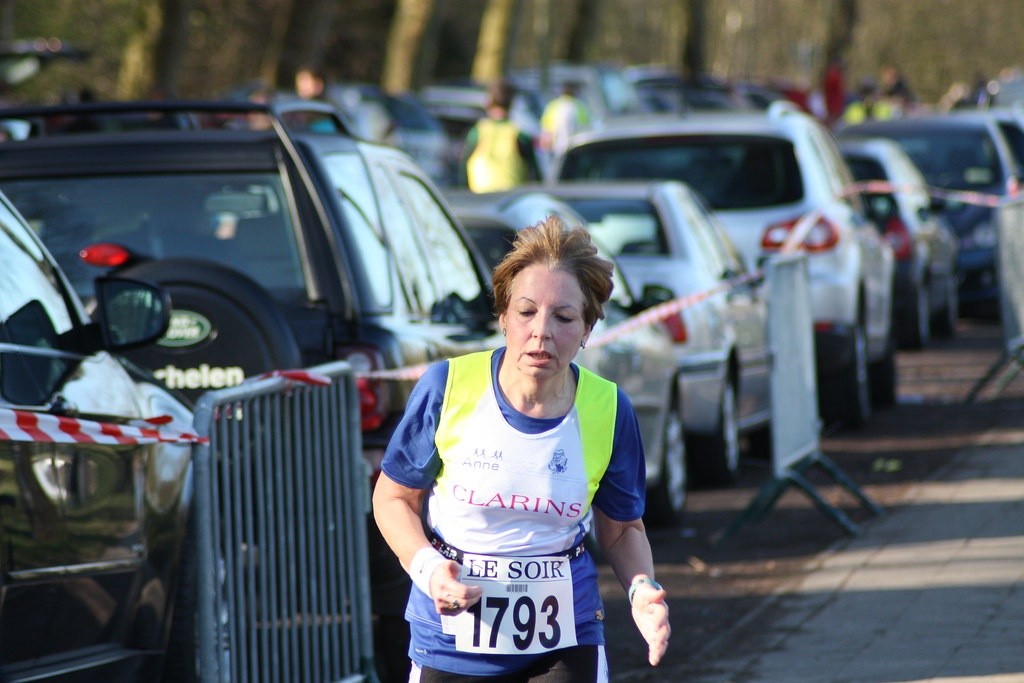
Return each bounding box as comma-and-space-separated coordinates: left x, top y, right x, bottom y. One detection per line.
0, 54, 1024, 615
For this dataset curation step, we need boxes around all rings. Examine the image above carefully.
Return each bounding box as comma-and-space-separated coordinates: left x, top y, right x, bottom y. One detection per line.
452, 598, 459, 609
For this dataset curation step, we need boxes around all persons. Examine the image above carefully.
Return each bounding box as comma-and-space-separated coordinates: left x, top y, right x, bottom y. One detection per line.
242, 67, 350, 135
458, 81, 588, 193
372, 215, 670, 683
829, 63, 996, 131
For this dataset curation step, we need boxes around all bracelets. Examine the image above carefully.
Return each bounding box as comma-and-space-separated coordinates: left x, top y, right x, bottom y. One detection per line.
410, 547, 445, 599
629, 579, 664, 606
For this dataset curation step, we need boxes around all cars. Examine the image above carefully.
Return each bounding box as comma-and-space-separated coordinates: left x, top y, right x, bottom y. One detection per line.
0, 181, 256, 683
